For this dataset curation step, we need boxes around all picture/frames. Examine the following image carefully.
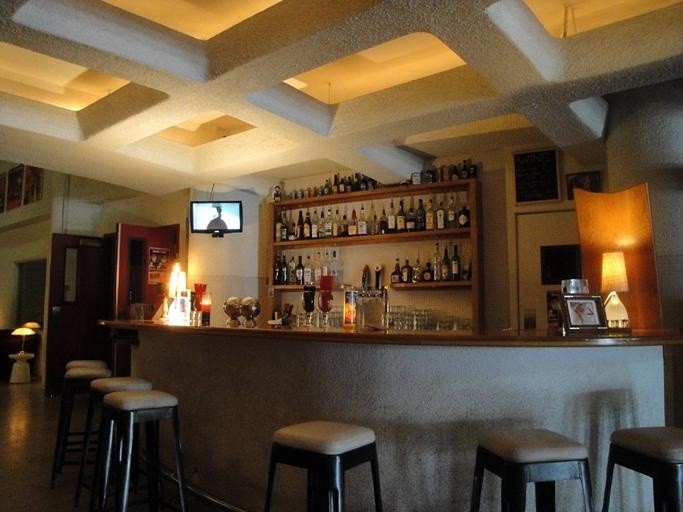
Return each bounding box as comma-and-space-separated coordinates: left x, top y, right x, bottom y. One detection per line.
562, 291, 608, 337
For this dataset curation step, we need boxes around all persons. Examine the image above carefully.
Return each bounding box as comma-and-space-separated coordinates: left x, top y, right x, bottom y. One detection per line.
205, 204, 229, 230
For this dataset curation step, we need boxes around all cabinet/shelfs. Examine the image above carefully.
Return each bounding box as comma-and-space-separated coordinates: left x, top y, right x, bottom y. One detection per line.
256, 177, 485, 338
94, 315, 683, 512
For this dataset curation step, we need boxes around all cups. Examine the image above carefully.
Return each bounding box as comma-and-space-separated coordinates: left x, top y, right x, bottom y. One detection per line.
130, 303, 137, 319
343, 285, 357, 328
190, 310, 203, 326
297, 314, 306, 327
136, 303, 144, 319
524, 308, 536, 328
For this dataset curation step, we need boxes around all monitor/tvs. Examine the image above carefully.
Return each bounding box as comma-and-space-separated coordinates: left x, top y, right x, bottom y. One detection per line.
540, 245, 581, 285
190, 201, 244, 238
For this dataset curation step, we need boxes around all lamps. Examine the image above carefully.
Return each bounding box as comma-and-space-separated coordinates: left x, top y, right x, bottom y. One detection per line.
598, 248, 631, 331
8, 326, 38, 384
164, 269, 190, 322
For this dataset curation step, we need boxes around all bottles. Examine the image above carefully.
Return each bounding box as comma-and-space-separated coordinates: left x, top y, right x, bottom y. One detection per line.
297, 210, 304, 240
295, 256, 302, 284
340, 215, 347, 236
315, 254, 321, 285
462, 160, 468, 179
325, 209, 333, 238
276, 207, 282, 241
458, 206, 471, 228
310, 186, 315, 196
303, 256, 315, 285
447, 197, 458, 228
334, 210, 340, 237
442, 249, 451, 281
424, 167, 450, 183
371, 214, 379, 235
357, 207, 367, 235
396, 200, 406, 232
304, 189, 308, 197
452, 245, 460, 281
450, 165, 460, 181
391, 258, 403, 283
333, 174, 339, 194
297, 189, 302, 199
281, 213, 287, 241
414, 259, 423, 281
324, 180, 329, 195
436, 202, 446, 230
422, 262, 434, 282
311, 210, 319, 238
406, 208, 416, 232
291, 190, 295, 200
318, 211, 326, 238
289, 256, 298, 284
281, 256, 288, 284
380, 208, 388, 234
274, 186, 281, 201
388, 201, 396, 233
411, 172, 421, 185
360, 182, 366, 190
330, 250, 340, 284
339, 178, 345, 192
434, 243, 442, 281
417, 199, 426, 231
425, 199, 436, 231
304, 212, 310, 239
201, 293, 210, 326
401, 259, 413, 282
347, 208, 358, 236
274, 256, 282, 284
463, 257, 472, 280
345, 176, 352, 192
368, 179, 373, 190
323, 252, 330, 276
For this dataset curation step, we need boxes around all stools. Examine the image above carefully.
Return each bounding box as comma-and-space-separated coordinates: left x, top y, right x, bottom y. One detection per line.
263, 420, 383, 512
65, 362, 108, 451
51, 367, 110, 486
602, 426, 683, 512
75, 376, 163, 504
92, 389, 186, 512
469, 428, 595, 512
